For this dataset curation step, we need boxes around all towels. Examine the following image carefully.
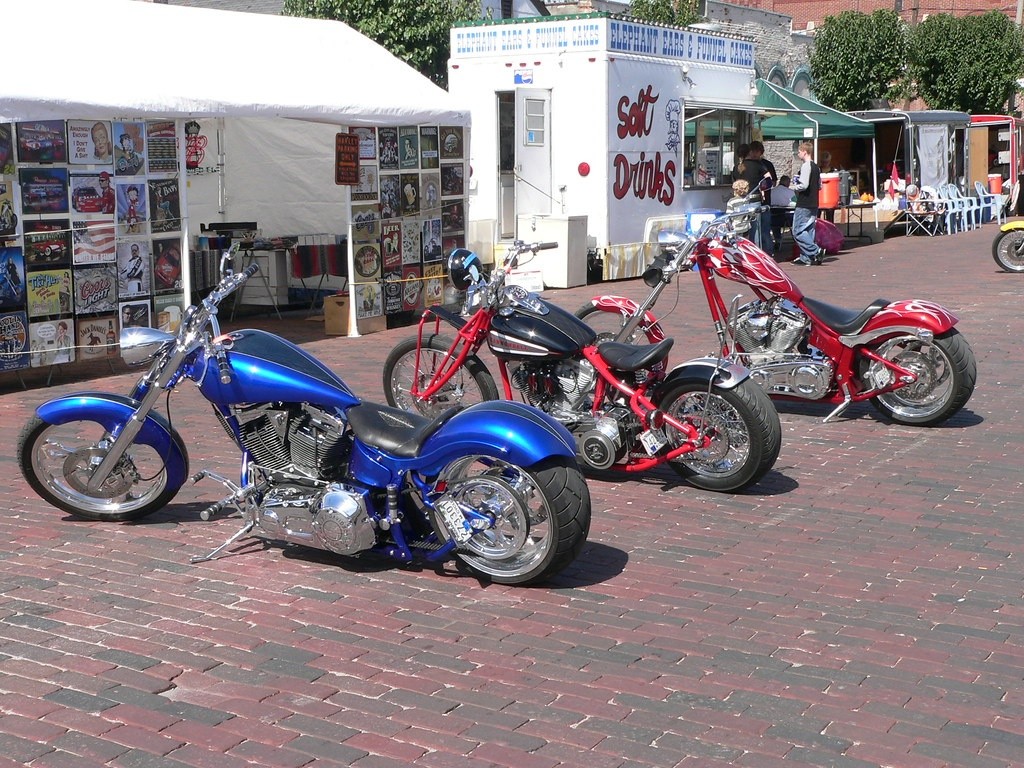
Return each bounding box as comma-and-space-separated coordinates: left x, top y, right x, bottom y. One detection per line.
189, 250, 223, 292
290, 244, 347, 279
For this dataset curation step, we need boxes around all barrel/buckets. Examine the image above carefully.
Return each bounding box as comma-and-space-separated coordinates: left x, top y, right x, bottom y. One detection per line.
988, 174, 1003, 195
829, 171, 849, 205
818, 172, 839, 209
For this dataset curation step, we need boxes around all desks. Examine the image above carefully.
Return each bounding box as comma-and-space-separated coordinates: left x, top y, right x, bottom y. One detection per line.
290, 243, 348, 316
772, 202, 877, 245
847, 168, 871, 194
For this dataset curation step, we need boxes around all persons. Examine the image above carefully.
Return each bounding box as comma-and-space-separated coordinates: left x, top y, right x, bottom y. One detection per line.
120, 244, 144, 293
727, 141, 795, 251
792, 142, 826, 266
121, 305, 132, 328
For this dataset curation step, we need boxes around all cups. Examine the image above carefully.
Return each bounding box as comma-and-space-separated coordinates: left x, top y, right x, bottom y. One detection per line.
793, 175, 799, 185
194, 235, 230, 251
710, 178, 715, 186
385, 243, 392, 257
404, 184, 416, 205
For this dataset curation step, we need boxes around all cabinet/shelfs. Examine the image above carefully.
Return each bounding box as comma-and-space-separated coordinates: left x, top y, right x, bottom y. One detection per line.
499, 169, 514, 238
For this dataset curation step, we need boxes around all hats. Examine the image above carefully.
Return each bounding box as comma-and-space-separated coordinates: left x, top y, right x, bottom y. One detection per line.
820, 151, 831, 161
157, 187, 161, 191
99, 171, 109, 180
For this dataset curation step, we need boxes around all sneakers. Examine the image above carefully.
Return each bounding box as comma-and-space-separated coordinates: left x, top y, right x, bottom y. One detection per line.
815, 248, 826, 265
790, 257, 810, 266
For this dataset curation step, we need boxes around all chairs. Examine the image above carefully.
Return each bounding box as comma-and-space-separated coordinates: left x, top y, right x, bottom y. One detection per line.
904, 181, 1007, 235
772, 214, 794, 253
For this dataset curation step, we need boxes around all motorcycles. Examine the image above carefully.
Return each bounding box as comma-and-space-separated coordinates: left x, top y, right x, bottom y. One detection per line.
16, 237, 592, 588
0, 264, 22, 305
574, 172, 979, 425
0, 200, 17, 232
991, 222, 1024, 275
382, 236, 783, 497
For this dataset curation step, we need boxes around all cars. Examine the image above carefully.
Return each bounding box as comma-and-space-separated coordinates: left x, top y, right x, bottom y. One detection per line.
20, 127, 64, 151
155, 247, 180, 285
31, 240, 68, 257
73, 185, 104, 214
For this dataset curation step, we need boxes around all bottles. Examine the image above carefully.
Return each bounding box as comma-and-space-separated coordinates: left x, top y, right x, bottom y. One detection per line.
107, 321, 115, 354
850, 194, 853, 205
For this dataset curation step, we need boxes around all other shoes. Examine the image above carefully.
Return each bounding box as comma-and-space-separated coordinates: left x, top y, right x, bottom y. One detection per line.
774, 242, 780, 249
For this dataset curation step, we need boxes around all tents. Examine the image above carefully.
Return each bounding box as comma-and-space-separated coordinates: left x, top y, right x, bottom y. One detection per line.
0, 1, 472, 338
684, 78, 1024, 236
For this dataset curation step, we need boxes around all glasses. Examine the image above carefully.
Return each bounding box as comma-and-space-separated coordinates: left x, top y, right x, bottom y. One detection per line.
124, 312, 131, 315
99, 179, 105, 182
132, 250, 137, 252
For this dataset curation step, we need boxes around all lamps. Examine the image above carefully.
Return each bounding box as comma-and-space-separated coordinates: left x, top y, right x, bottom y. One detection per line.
680, 65, 698, 90
750, 81, 760, 96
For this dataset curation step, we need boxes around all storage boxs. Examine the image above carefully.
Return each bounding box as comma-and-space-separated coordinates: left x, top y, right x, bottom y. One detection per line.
234, 249, 289, 306
323, 293, 387, 336
298, 233, 346, 245
491, 267, 544, 293
684, 208, 725, 233
899, 198, 910, 210
885, 192, 901, 198
